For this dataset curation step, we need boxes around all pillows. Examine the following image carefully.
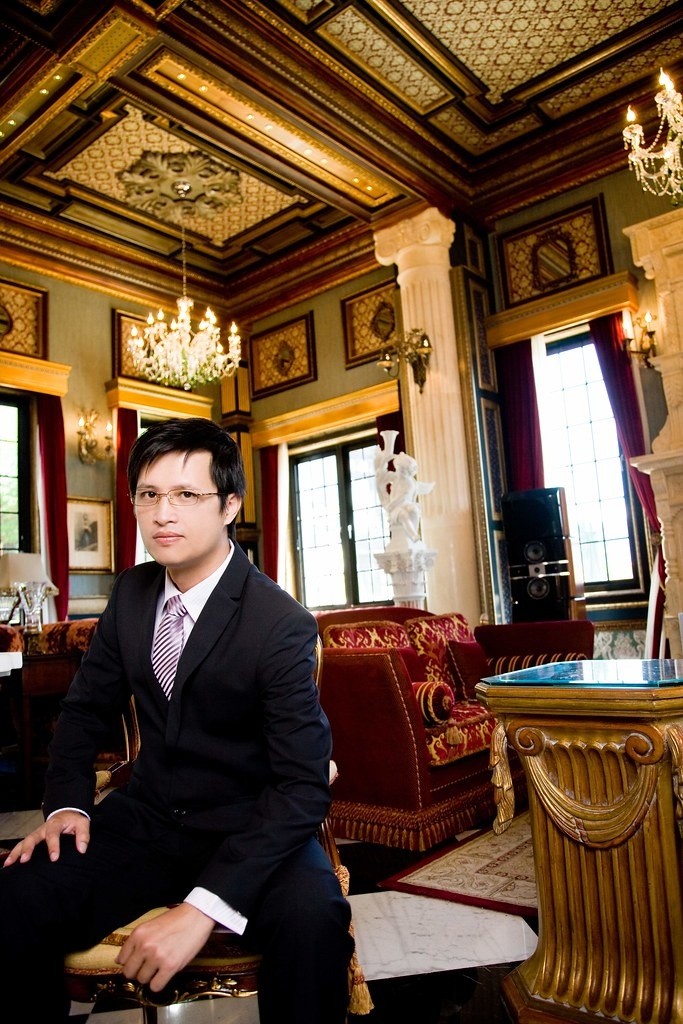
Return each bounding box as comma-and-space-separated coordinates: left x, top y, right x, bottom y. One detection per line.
394, 645, 428, 684
445, 638, 494, 700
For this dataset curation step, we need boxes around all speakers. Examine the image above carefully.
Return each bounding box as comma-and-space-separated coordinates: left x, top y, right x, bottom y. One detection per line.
502, 487, 586, 622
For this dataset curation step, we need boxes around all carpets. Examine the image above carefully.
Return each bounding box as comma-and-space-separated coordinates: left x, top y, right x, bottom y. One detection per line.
374, 801, 537, 919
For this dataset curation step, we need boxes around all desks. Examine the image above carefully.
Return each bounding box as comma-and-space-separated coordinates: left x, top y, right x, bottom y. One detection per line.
473, 660, 683, 1024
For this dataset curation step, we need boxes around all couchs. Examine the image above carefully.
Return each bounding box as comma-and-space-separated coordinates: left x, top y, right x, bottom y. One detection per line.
305, 605, 594, 854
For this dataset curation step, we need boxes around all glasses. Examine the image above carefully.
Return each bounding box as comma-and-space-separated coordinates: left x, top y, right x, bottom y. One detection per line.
126, 487, 226, 505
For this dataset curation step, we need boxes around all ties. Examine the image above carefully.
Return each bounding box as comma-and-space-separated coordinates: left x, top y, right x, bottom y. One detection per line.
153, 596, 189, 700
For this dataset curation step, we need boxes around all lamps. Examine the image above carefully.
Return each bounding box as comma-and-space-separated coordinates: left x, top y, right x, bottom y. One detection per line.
376, 327, 433, 396
0, 551, 61, 631
76, 411, 116, 466
623, 66, 683, 204
620, 309, 659, 369
126, 148, 242, 394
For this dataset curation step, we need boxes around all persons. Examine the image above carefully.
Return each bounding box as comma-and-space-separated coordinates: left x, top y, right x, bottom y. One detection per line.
372, 429, 434, 552
0, 418, 350, 1024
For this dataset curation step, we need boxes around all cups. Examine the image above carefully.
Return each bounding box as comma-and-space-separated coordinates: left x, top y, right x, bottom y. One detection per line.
14, 581, 48, 633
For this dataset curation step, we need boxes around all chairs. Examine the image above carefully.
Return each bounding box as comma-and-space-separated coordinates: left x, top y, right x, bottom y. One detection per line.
59, 625, 375, 1024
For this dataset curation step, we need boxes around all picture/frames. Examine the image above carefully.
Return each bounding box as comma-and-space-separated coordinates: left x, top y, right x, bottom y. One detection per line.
66, 495, 116, 576
